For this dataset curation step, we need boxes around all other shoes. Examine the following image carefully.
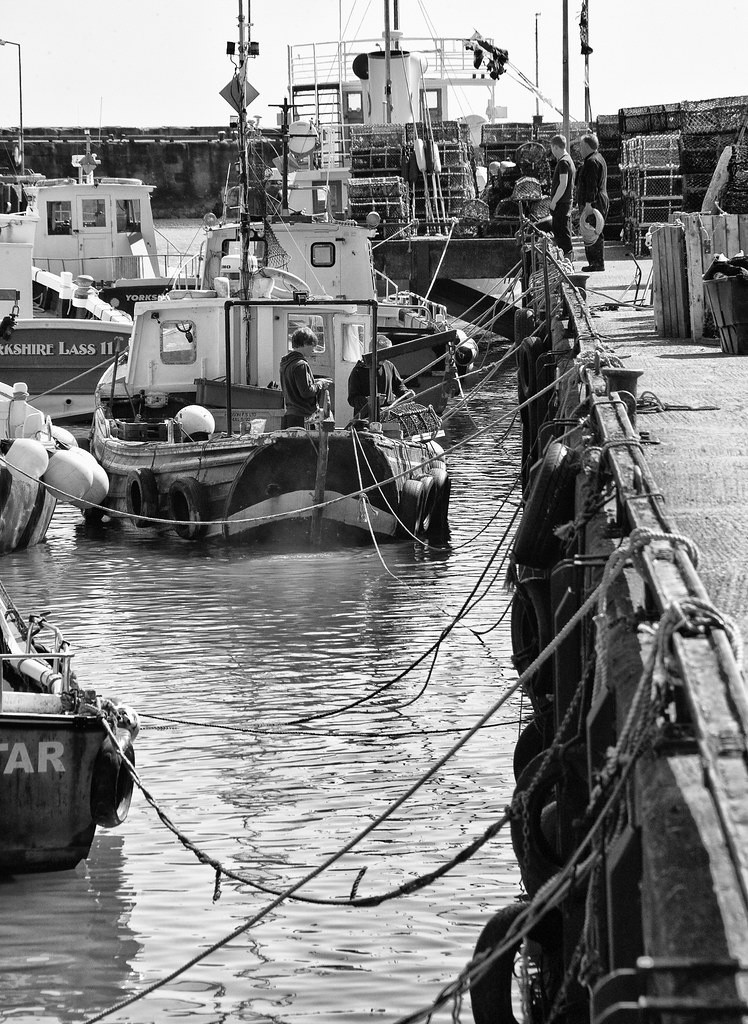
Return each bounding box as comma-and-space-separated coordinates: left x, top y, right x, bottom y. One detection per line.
582, 265, 605, 272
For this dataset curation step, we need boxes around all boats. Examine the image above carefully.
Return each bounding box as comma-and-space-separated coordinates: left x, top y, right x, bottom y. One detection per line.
2, 567, 111, 883
270, 50, 525, 356
0, 385, 63, 555
0, 165, 206, 425
80, 216, 454, 546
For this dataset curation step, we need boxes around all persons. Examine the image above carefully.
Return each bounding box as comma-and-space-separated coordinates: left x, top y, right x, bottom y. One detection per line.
348, 334, 416, 419
575, 133, 610, 272
280, 326, 333, 430
549, 134, 576, 255
266, 180, 282, 215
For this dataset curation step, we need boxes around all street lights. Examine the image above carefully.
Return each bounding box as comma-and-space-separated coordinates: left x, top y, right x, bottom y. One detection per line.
0, 38, 25, 178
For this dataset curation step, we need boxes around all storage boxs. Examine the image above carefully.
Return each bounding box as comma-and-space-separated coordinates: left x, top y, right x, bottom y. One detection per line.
702, 275, 748, 326
719, 323, 748, 355
